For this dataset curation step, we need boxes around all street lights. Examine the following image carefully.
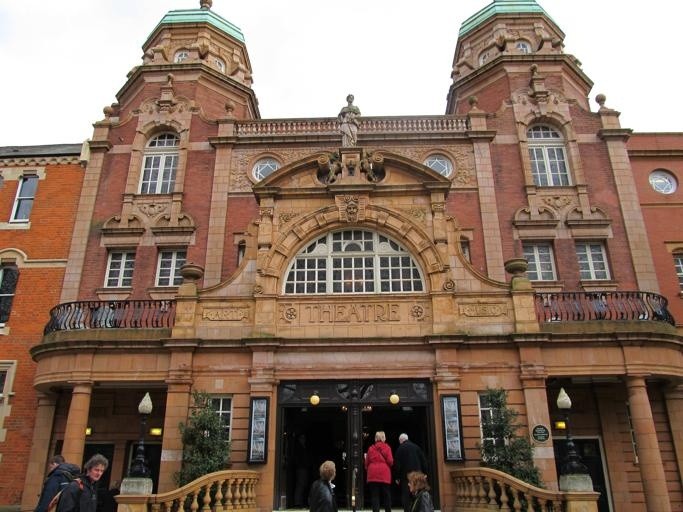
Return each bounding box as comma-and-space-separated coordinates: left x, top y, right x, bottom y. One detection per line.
556, 387, 587, 473
128, 392, 152, 478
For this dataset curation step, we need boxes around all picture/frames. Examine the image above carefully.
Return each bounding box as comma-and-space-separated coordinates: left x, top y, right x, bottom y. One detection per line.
440, 394, 465, 464
247, 396, 270, 464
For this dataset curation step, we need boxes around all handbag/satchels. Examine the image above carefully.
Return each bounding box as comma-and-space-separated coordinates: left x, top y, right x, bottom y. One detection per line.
47, 487, 67, 512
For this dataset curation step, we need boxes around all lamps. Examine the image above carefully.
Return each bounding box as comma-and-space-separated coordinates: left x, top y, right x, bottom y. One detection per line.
137, 391, 156, 415
389, 390, 402, 405
308, 390, 322, 405
556, 387, 573, 410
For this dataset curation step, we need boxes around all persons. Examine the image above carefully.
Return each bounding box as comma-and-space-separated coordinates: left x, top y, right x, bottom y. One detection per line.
364, 430, 393, 511
308, 460, 337, 511
393, 432, 429, 512
336, 94, 362, 149
252, 400, 264, 459
404, 471, 434, 511
34, 454, 80, 511
55, 453, 109, 511
